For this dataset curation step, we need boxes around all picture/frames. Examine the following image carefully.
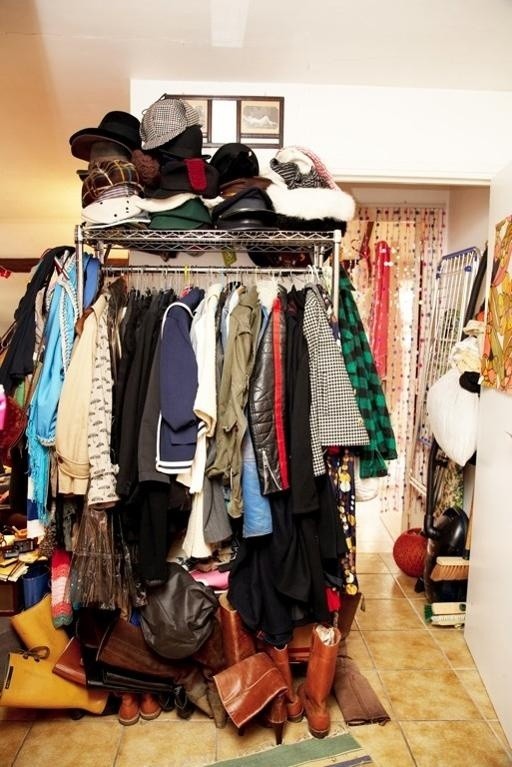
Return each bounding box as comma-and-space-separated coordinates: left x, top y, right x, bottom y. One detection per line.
165, 93, 286, 148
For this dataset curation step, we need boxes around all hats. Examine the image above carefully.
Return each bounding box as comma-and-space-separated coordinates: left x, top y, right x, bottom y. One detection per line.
69, 96, 276, 232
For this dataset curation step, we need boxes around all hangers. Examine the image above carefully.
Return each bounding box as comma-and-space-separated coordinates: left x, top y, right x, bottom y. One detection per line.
102, 261, 333, 312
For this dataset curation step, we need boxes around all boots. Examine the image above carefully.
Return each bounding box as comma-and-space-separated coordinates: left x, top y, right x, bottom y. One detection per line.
0, 573, 305, 746
332, 654, 391, 727
298, 621, 342, 739
